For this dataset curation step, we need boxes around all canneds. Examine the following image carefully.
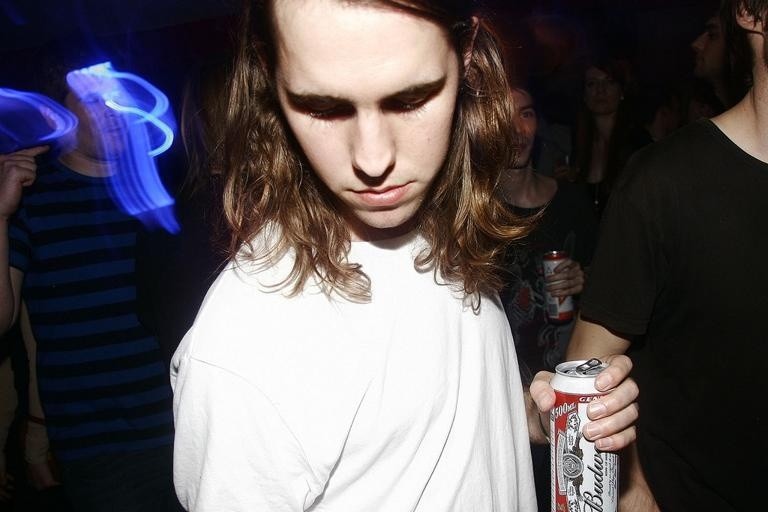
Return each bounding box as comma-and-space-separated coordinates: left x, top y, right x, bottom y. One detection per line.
544, 251, 575, 321
549, 359, 619, 512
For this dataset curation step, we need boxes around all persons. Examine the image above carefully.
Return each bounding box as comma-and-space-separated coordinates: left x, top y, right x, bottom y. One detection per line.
169, 0, 640, 511
564, 0, 768, 512
690, 11, 751, 115
1, 299, 76, 511
488, 72, 602, 512
687, 91, 723, 121
570, 55, 653, 213
0, 54, 185, 511
626, 80, 681, 152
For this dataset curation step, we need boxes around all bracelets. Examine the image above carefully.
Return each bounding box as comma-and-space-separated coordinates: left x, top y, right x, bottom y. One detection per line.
538, 409, 549, 441
